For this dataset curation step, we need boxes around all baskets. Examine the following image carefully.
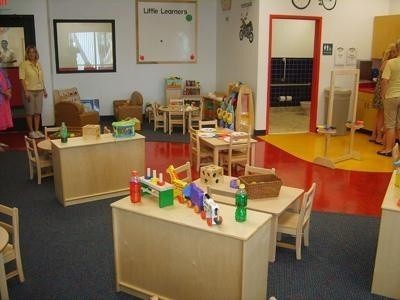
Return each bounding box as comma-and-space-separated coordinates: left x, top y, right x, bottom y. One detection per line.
239, 173, 282, 198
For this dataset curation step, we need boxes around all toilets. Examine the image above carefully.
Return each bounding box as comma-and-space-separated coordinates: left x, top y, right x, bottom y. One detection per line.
300, 101, 311, 115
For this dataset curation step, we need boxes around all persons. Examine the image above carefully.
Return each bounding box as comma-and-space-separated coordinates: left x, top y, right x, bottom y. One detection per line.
377, 39, 400, 157
19, 46, 48, 139
369, 43, 397, 146
0, 40, 17, 64
0, 68, 14, 147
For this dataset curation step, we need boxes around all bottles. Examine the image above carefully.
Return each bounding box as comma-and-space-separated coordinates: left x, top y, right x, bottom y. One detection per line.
61, 122, 68, 143
128, 169, 143, 205
234, 183, 249, 224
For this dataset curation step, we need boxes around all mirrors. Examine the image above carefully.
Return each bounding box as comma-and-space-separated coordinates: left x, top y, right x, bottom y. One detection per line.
53, 19, 116, 73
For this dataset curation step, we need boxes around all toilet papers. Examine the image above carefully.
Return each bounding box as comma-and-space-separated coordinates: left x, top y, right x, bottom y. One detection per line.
286, 96, 292, 101
279, 96, 285, 102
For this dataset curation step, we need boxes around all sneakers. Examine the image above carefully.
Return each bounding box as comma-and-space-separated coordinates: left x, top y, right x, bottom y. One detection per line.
377, 151, 392, 156
36, 131, 44, 137
29, 131, 39, 138
375, 140, 384, 145
369, 139, 375, 142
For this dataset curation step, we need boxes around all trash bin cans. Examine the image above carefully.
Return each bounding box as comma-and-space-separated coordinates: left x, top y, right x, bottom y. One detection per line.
324, 87, 351, 135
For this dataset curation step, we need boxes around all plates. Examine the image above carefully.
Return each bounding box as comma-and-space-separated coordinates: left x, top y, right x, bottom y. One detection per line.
201, 128, 247, 144
